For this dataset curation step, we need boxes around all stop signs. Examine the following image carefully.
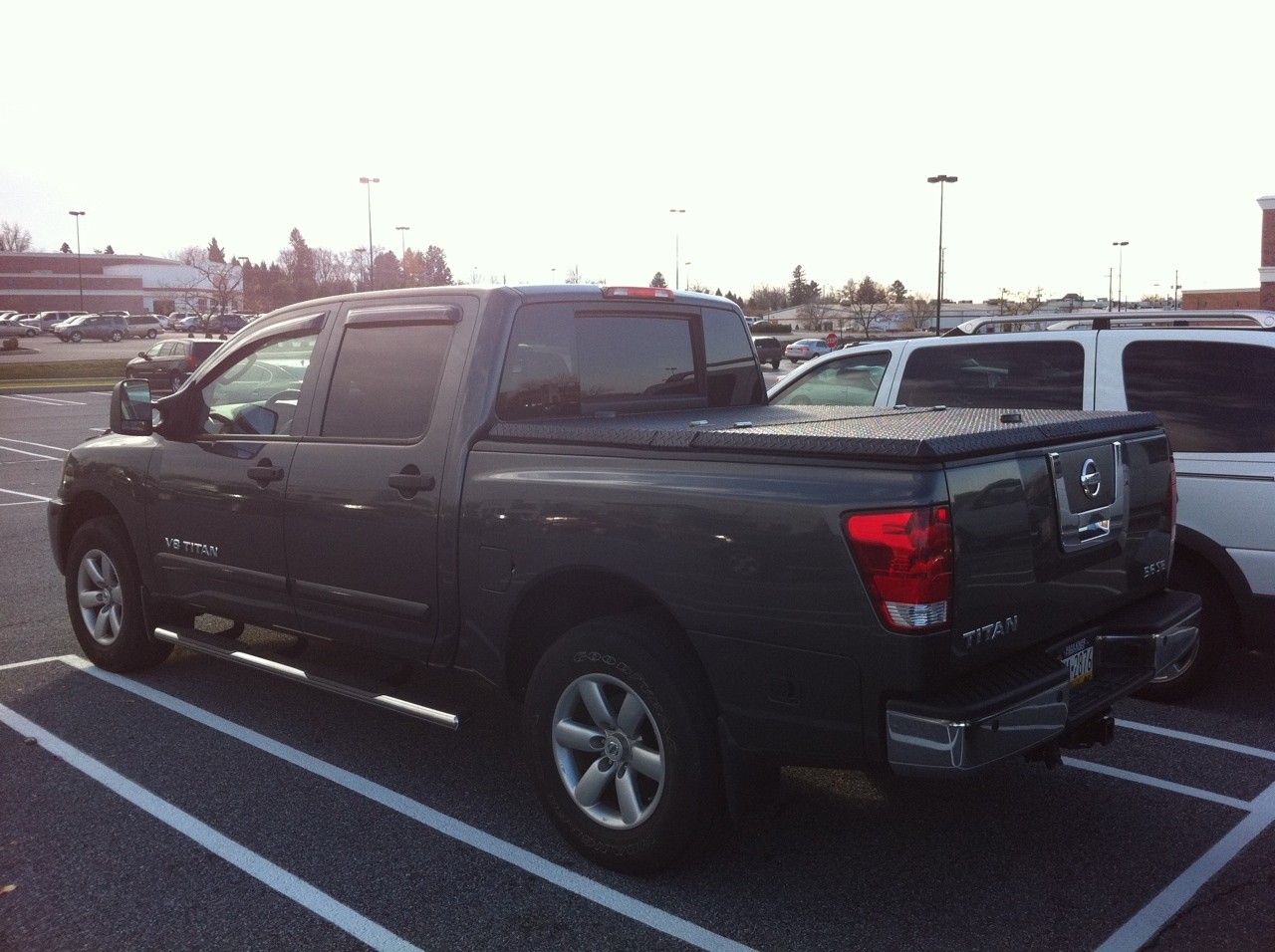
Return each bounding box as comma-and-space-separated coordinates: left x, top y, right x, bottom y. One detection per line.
825, 333, 838, 349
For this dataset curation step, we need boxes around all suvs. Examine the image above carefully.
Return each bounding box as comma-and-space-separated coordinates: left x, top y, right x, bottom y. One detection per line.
751, 336, 783, 369
764, 306, 1275, 704
125, 337, 227, 394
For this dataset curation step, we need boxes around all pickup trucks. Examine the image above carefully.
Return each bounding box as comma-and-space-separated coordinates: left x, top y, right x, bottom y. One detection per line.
46, 284, 1206, 878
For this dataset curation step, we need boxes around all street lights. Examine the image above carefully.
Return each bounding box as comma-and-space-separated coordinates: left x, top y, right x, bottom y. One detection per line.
472, 267, 478, 285
685, 262, 692, 291
551, 268, 557, 285
1112, 240, 1130, 328
69, 210, 86, 311
358, 176, 381, 291
669, 208, 686, 290
355, 248, 368, 291
396, 226, 411, 288
926, 174, 959, 336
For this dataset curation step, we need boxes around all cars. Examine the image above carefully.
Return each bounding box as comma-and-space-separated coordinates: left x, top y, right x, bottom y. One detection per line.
784, 338, 836, 364
0, 310, 267, 343
744, 316, 771, 329
